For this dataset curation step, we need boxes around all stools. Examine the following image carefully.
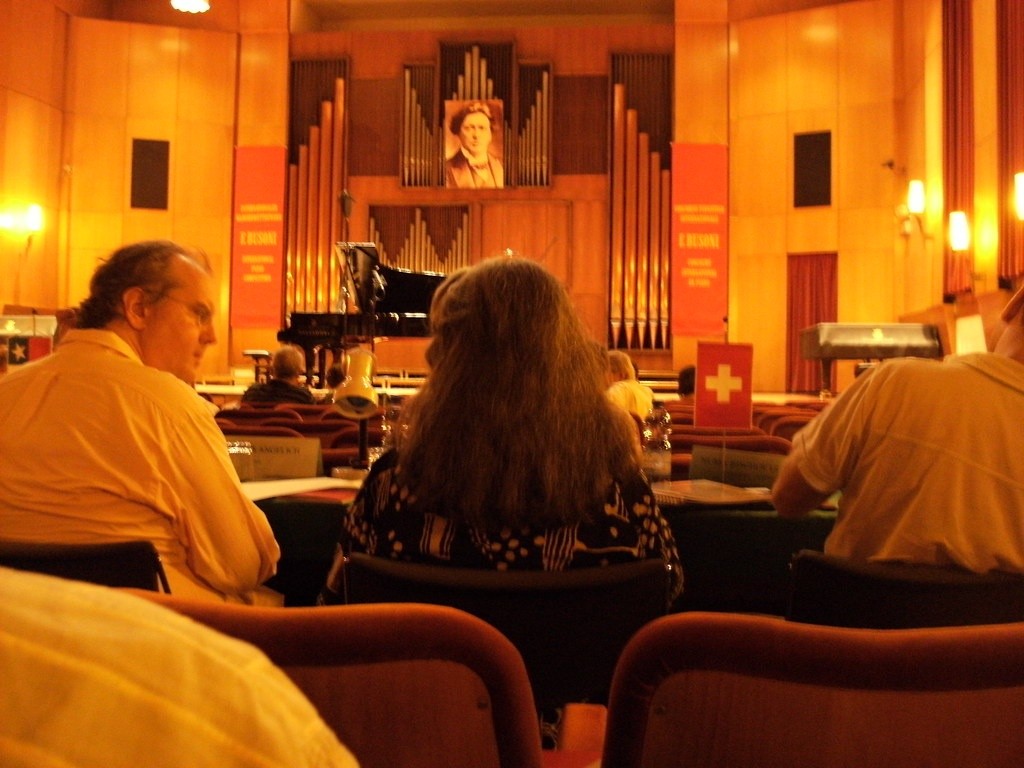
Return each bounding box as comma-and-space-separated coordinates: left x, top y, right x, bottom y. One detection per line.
244, 349, 275, 386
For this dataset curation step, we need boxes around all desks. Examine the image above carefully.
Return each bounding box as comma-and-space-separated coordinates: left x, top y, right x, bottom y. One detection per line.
253, 488, 838, 615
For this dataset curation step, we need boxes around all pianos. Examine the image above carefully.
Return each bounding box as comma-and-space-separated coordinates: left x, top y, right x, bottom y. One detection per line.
276, 242, 451, 388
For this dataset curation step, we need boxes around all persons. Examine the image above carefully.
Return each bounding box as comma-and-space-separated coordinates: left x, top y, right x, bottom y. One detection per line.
48, 306, 80, 353
0, 567, 360, 768
324, 249, 686, 608
0, 240, 283, 605
322, 365, 344, 403
602, 350, 656, 422
242, 343, 316, 404
670, 366, 694, 407
447, 104, 503, 189
764, 273, 1024, 579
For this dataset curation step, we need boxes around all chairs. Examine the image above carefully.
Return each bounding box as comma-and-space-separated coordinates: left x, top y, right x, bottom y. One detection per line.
1, 539, 174, 591
786, 547, 1023, 629
648, 394, 839, 485
200, 390, 409, 477
112, 587, 542, 767
559, 610, 1023, 767
329, 549, 676, 749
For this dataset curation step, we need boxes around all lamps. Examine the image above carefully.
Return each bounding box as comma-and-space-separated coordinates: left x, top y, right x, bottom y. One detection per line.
907, 179, 933, 247
949, 209, 985, 282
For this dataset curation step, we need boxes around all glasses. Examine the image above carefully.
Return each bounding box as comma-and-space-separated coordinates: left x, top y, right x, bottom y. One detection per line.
119, 285, 211, 330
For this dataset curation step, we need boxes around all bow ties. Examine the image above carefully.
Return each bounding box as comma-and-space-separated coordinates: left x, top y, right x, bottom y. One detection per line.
475, 164, 488, 169
